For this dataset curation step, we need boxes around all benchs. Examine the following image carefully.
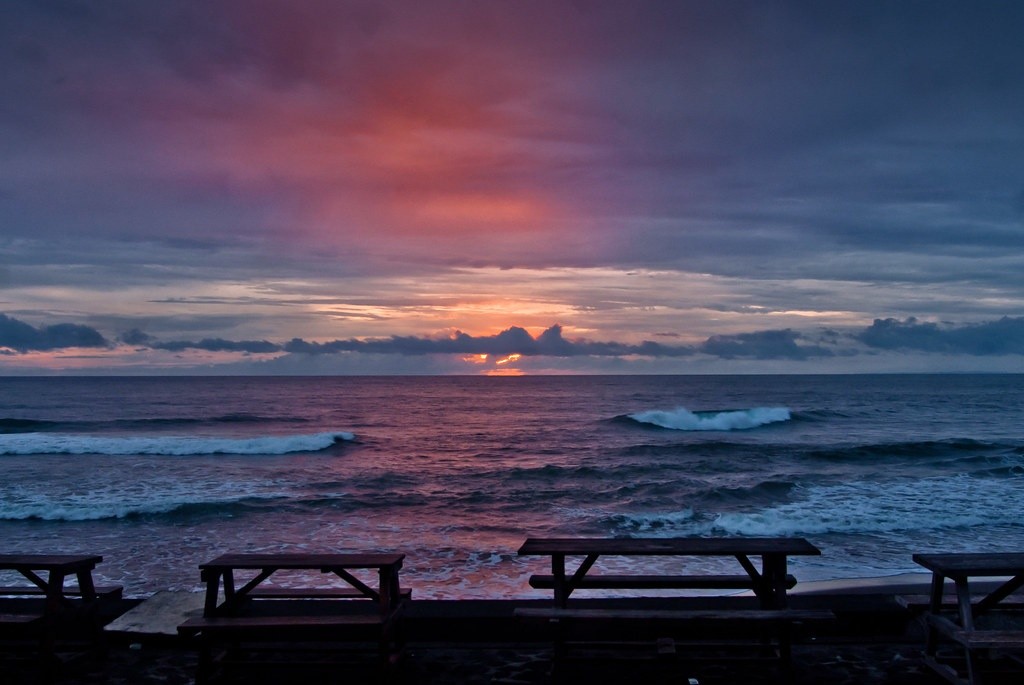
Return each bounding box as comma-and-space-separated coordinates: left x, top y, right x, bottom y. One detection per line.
176, 615, 388, 681
894, 592, 1024, 662
237, 587, 412, 622
2, 586, 124, 623
528, 572, 799, 609
513, 607, 834, 679
953, 627, 1024, 685
0, 612, 44, 663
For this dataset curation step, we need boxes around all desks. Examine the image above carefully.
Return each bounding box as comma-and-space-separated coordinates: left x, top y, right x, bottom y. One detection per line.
198, 554, 407, 661
913, 548, 1024, 685
519, 537, 822, 678
0, 554, 102, 657
